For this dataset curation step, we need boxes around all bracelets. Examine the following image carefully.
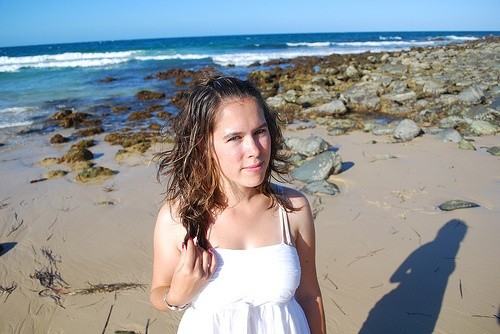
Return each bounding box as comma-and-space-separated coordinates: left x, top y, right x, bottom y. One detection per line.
164, 290, 192, 312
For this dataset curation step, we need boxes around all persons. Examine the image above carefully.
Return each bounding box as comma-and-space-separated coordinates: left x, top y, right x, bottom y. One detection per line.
152, 74, 327, 334
